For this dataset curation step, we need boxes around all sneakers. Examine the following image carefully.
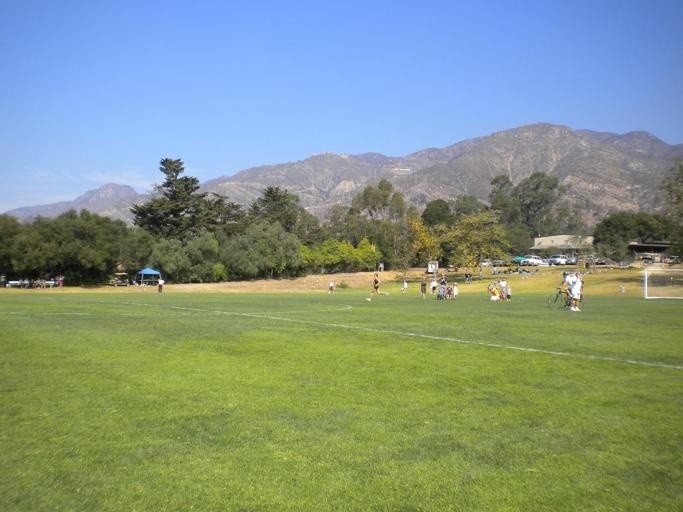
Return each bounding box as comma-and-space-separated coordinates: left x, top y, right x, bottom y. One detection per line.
569, 305, 581, 312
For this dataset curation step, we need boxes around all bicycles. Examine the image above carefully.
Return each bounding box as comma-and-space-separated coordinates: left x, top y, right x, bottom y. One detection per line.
547, 287, 588, 310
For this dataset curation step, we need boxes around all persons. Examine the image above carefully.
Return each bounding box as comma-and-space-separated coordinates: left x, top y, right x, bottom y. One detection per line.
465, 272, 473, 285
617, 285, 625, 293
420, 275, 427, 298
507, 267, 513, 278
400, 279, 408, 293
328, 279, 336, 295
558, 271, 584, 313
366, 273, 389, 302
1, 273, 6, 288
18, 274, 64, 288
479, 269, 483, 282
133, 275, 165, 293
487, 280, 512, 304
430, 273, 458, 304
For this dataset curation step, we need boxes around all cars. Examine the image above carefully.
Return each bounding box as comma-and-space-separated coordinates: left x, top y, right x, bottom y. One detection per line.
477, 250, 681, 267
